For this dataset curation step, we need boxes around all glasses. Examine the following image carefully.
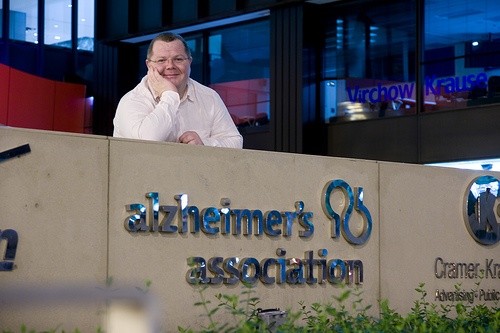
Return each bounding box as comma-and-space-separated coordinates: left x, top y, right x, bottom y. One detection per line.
147, 56, 189, 67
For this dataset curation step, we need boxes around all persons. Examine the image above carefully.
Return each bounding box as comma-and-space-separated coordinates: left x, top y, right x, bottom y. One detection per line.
112, 32, 244, 150
